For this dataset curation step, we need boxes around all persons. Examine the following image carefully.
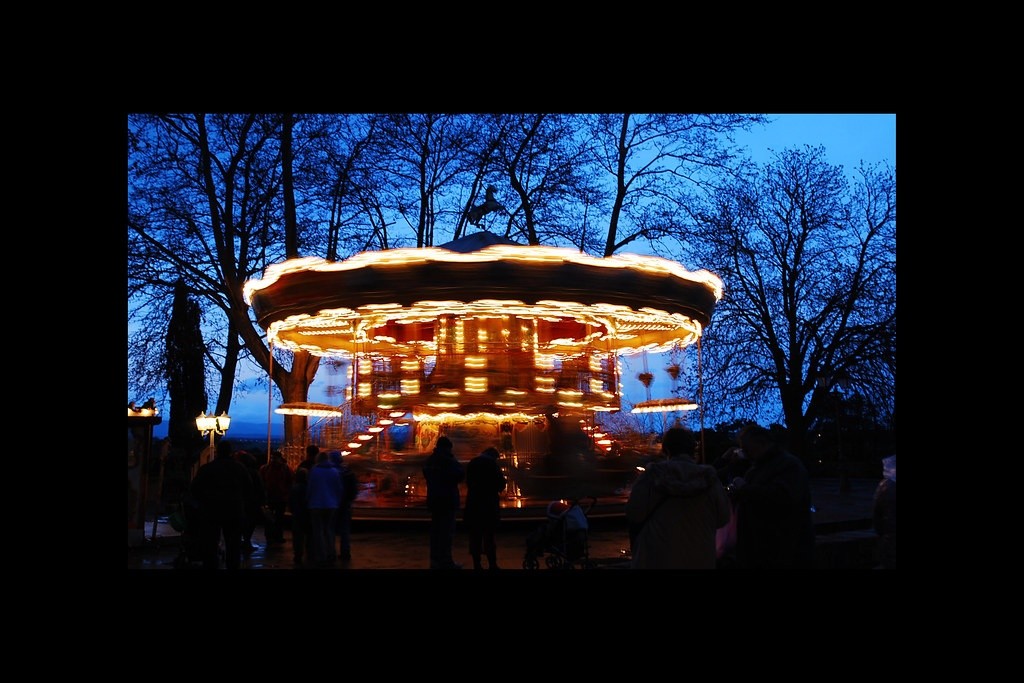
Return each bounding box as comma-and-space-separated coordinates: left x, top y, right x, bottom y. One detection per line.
422, 438, 467, 571
304, 453, 343, 570
289, 467, 318, 563
735, 432, 815, 573
257, 452, 296, 544
329, 451, 357, 563
297, 444, 320, 475
186, 441, 254, 574
626, 426, 732, 571
236, 455, 266, 555
541, 489, 591, 572
711, 425, 772, 483
466, 449, 506, 576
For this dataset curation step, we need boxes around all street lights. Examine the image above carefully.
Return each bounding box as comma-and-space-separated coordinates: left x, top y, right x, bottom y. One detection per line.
195, 410, 231, 463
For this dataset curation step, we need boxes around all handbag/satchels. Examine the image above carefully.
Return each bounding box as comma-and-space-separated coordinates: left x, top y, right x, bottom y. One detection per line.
627, 521, 647, 553
